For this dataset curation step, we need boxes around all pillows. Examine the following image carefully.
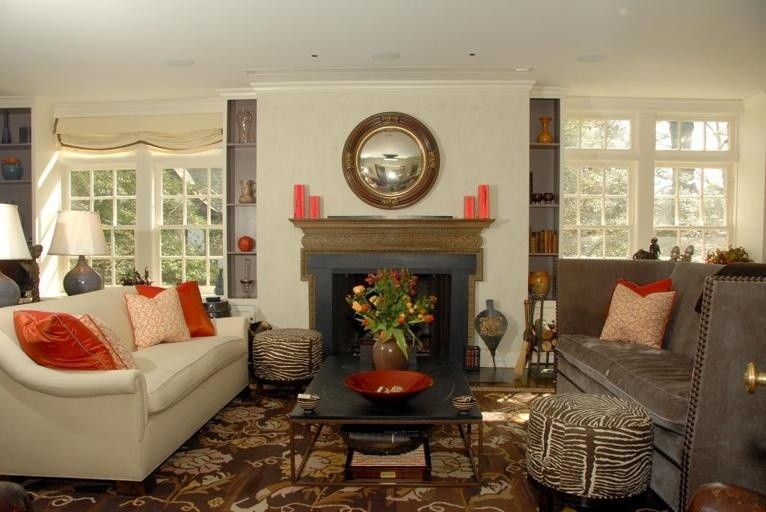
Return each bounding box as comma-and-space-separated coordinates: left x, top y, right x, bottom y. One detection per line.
70, 310, 141, 371
122, 283, 195, 352
133, 276, 217, 339
603, 275, 677, 322
597, 283, 679, 351
9, 306, 118, 373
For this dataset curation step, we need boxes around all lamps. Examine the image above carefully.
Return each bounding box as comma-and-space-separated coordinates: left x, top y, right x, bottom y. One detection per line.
0, 201, 34, 312
45, 209, 112, 297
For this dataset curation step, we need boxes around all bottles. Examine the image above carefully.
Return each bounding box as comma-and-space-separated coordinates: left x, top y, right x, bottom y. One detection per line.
214, 269, 223, 294
241, 258, 253, 299
1, 108, 10, 145
238, 110, 254, 143
536, 117, 554, 143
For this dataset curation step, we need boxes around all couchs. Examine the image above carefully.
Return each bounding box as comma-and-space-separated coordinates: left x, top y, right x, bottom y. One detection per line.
550, 251, 766, 511
0, 283, 254, 500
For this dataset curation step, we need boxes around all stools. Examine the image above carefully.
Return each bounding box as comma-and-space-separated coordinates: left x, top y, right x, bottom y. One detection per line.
680, 475, 766, 512
250, 326, 326, 405
515, 391, 662, 512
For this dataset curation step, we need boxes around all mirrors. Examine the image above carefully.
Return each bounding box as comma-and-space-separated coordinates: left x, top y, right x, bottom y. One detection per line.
340, 106, 442, 209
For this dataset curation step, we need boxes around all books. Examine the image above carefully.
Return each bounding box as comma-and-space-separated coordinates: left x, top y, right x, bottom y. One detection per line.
530, 229, 556, 253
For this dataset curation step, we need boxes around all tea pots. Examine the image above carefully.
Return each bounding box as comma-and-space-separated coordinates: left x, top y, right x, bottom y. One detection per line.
238, 179, 256, 203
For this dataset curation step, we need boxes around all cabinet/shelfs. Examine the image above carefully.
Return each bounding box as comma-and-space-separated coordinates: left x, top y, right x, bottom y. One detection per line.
224, 97, 257, 324
0, 104, 32, 258
527, 97, 564, 301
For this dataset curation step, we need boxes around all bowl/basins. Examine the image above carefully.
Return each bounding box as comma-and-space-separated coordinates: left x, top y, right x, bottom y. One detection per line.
452, 395, 478, 413
298, 394, 321, 414
342, 369, 435, 407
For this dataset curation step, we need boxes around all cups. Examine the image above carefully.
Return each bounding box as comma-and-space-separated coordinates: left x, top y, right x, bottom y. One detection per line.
532, 192, 554, 205
293, 185, 305, 218
478, 183, 489, 218
528, 270, 551, 300
530, 226, 558, 253
18, 128, 28, 144
463, 196, 475, 220
308, 196, 320, 219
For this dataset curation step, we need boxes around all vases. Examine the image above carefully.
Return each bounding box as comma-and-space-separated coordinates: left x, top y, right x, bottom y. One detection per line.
535, 114, 555, 145
528, 271, 554, 302
236, 175, 256, 205
233, 106, 255, 145
236, 255, 255, 300
1, 111, 11, 144
371, 334, 409, 371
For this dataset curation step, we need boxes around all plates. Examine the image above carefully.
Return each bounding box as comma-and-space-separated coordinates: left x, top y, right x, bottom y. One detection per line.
206, 297, 220, 302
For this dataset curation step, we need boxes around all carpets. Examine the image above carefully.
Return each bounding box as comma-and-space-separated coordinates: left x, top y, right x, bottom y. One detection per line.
15, 377, 677, 510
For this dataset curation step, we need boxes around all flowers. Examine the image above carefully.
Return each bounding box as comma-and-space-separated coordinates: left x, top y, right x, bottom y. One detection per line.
340, 264, 440, 362
